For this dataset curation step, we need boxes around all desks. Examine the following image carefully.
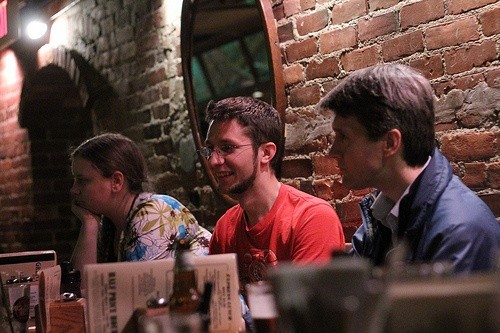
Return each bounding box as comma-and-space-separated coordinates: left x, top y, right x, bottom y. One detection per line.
28, 300, 85, 333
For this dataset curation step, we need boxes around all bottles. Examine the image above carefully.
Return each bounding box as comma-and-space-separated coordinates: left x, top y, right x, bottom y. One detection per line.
69, 266, 83, 297
245, 280, 279, 333
168, 239, 204, 333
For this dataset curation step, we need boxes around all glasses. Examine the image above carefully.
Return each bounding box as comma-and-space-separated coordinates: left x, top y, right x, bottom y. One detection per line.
199, 143, 253, 157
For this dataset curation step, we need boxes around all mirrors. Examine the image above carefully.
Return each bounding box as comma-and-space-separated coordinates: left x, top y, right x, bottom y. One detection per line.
179, 0, 286, 207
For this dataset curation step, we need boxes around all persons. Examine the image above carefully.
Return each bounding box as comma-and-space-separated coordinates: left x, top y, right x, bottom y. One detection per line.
204, 97, 346, 295
318, 64, 500, 277
68, 133, 269, 333
